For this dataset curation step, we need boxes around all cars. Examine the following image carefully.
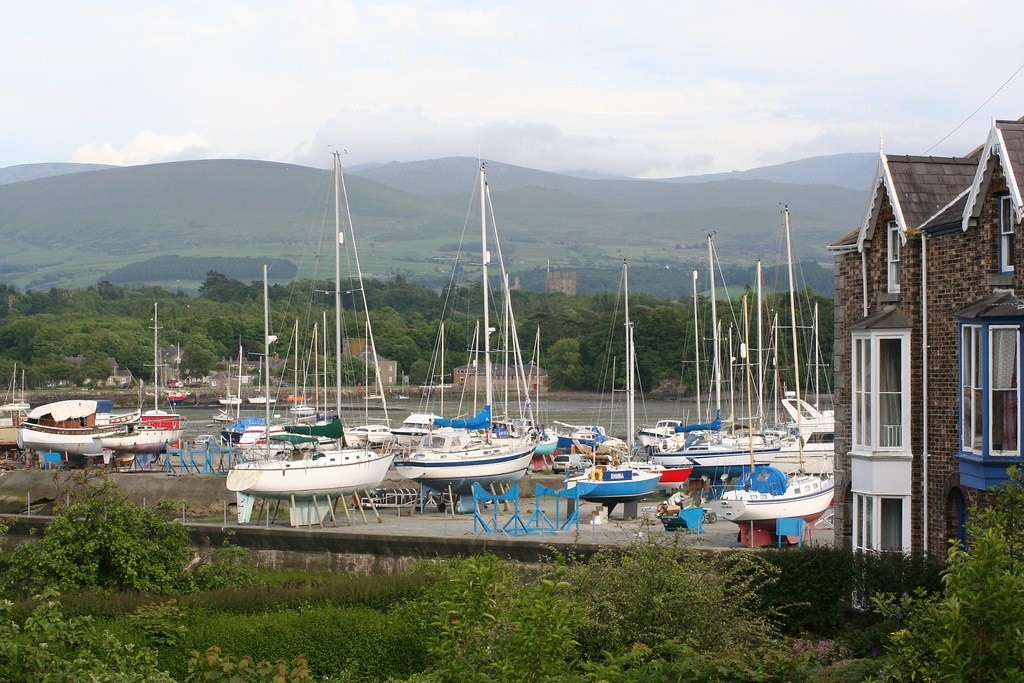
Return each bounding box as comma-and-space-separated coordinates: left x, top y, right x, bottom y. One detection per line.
168, 381, 184, 387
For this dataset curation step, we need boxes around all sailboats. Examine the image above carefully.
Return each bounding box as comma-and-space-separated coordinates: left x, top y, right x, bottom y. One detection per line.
0, 142, 834, 524
